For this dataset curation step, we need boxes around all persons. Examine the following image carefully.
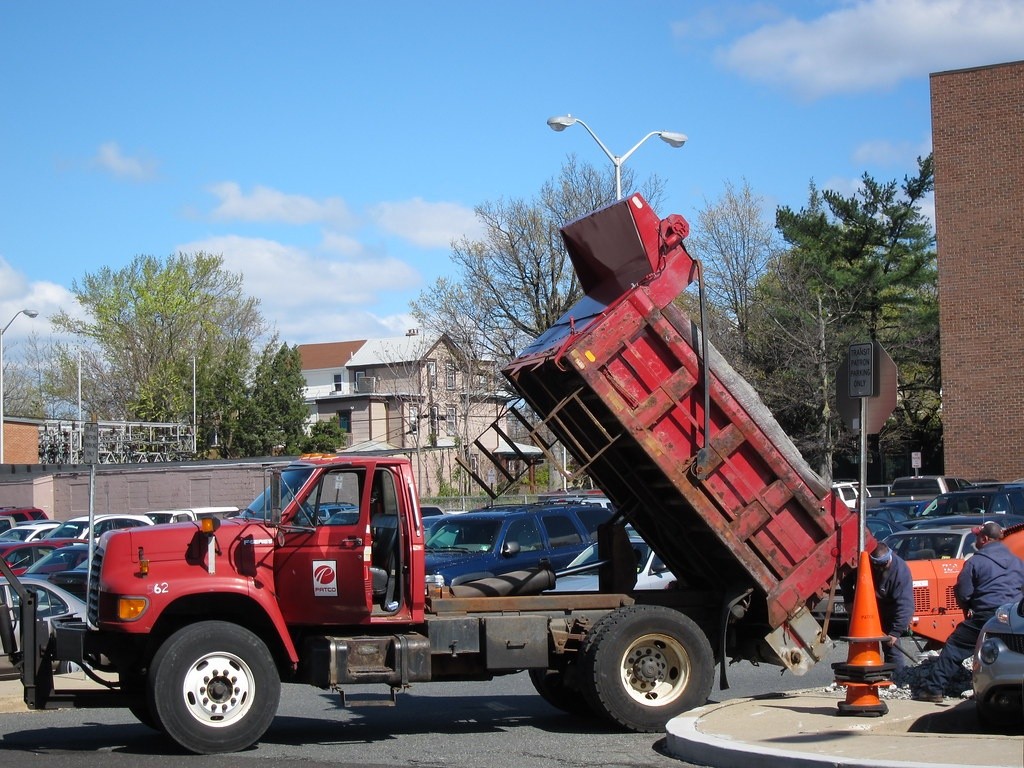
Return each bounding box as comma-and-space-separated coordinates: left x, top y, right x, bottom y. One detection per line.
917, 521, 1024, 703
839, 542, 915, 670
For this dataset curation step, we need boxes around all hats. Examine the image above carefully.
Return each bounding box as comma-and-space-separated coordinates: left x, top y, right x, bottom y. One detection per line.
971, 520, 1004, 540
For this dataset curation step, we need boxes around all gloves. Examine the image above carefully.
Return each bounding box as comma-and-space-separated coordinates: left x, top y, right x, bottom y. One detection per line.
885, 635, 897, 648
844, 601, 853, 612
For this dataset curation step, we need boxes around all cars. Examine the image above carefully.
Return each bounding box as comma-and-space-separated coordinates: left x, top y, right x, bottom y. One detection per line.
0, 507, 240, 606
299, 503, 455, 542
547, 532, 676, 591
865, 478, 1024, 560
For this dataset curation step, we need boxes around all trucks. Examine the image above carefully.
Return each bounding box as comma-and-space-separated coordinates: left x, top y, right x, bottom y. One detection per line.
20, 194, 877, 758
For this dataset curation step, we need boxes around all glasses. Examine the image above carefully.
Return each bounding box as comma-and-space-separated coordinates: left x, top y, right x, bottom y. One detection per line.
873, 560, 889, 567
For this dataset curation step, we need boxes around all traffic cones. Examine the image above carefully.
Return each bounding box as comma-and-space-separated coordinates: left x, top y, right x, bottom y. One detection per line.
833, 550, 895, 721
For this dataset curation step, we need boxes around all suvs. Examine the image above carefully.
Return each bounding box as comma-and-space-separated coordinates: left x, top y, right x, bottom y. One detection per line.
423, 503, 631, 588
832, 480, 871, 507
887, 479, 971, 503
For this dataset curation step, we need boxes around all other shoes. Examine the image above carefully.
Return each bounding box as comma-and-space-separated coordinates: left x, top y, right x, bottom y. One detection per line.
917, 689, 943, 702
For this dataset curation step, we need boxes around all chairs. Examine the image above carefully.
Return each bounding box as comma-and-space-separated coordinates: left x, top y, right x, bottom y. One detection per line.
369, 514, 399, 603
915, 549, 936, 560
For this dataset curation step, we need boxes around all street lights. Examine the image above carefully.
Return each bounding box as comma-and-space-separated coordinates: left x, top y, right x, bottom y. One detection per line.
0, 308, 38, 464
187, 357, 197, 454
545, 111, 687, 199
